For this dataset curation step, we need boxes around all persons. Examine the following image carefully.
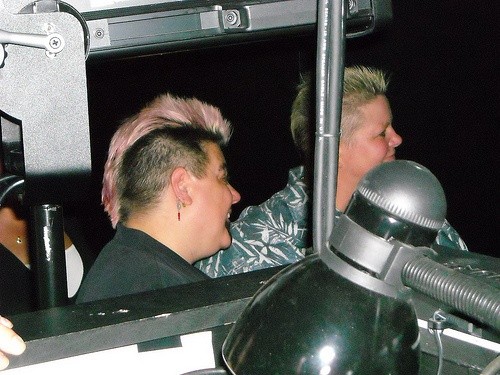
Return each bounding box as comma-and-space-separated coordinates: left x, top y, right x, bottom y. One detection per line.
75, 93, 242, 303
0, 142, 84, 371
193, 68, 469, 279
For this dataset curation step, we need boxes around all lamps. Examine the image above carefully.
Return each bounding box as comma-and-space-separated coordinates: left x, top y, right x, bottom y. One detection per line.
221, 159, 500, 375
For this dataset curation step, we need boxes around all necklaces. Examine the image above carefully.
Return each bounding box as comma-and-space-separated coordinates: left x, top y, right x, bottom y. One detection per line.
17, 234, 23, 244
